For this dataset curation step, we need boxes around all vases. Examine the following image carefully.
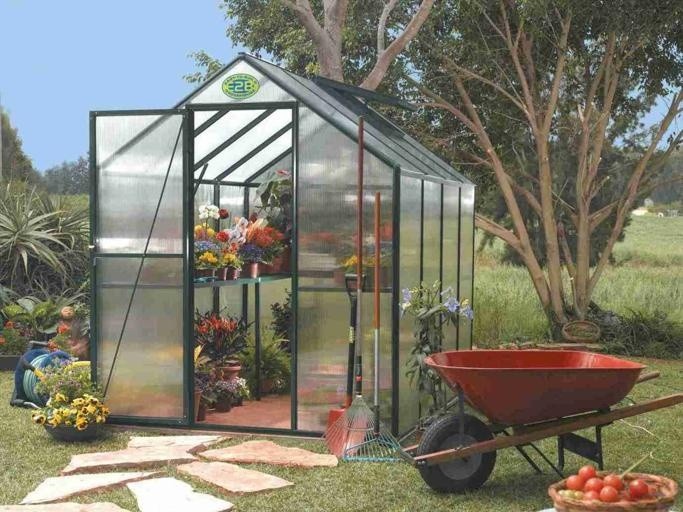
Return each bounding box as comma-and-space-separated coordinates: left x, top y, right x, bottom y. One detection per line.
220, 364, 242, 382
194, 389, 203, 422
42, 423, 99, 441
333, 266, 388, 289
215, 398, 230, 412
195, 248, 290, 279
0, 356, 20, 372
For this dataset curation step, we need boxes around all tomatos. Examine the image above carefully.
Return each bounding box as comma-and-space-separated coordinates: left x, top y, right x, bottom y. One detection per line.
585, 490, 599, 502
578, 465, 597, 480
584, 477, 605, 491
628, 480, 647, 499
599, 485, 616, 500
566, 477, 587, 491
606, 476, 626, 490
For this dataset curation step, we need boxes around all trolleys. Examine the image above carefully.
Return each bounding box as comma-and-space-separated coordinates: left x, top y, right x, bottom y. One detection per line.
400, 348, 681, 495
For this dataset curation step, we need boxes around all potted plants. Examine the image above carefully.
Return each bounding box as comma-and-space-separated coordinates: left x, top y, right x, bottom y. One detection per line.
239, 323, 290, 395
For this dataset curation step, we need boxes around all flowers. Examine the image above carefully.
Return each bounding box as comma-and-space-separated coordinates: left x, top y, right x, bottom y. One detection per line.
29, 356, 111, 433
193, 309, 250, 366
192, 169, 295, 269
340, 255, 384, 273
0, 321, 34, 355
398, 280, 474, 431
216, 378, 246, 399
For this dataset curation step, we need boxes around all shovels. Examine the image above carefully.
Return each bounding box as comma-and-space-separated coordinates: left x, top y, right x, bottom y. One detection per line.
329, 274, 369, 457
